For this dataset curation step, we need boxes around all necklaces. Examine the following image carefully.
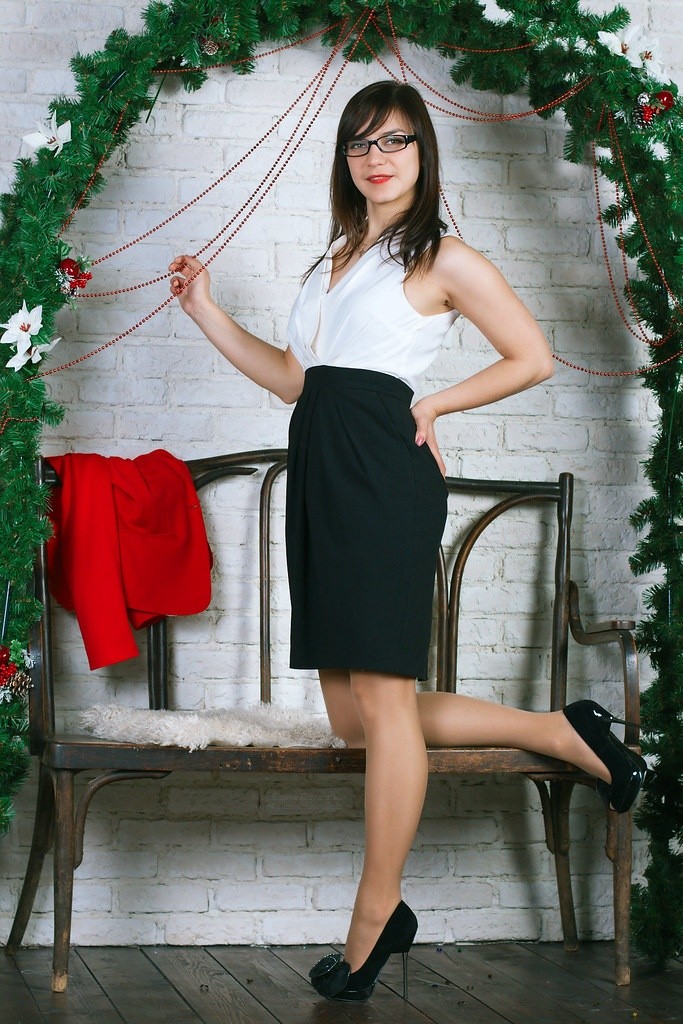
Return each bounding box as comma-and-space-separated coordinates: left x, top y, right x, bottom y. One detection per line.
358, 248, 365, 257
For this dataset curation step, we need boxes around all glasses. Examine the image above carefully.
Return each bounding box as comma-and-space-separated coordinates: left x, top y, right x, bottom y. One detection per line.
338, 134, 419, 157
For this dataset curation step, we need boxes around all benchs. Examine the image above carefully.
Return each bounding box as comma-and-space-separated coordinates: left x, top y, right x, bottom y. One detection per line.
6, 444, 645, 993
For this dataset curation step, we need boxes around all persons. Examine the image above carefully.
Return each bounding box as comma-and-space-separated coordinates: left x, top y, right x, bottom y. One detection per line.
170, 81, 646, 1000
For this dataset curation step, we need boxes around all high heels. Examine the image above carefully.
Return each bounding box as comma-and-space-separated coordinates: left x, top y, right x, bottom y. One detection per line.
308, 900, 419, 1003
562, 699, 648, 816
